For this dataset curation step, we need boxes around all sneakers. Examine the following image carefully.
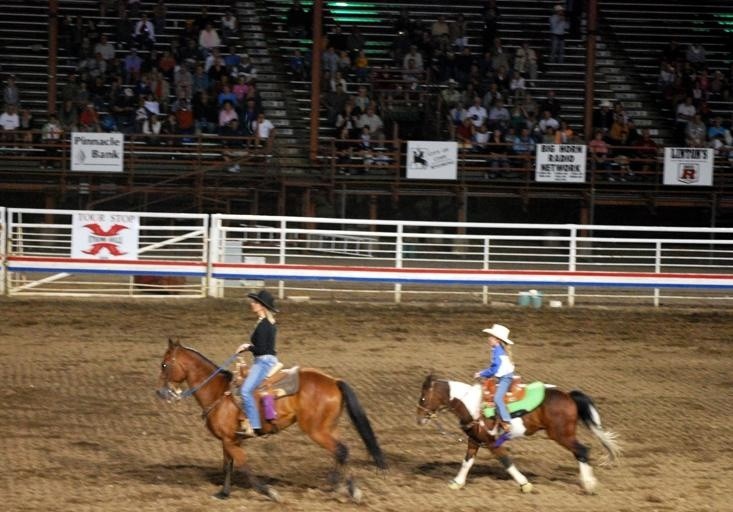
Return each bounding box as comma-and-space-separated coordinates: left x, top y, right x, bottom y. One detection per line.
498, 421, 511, 436
234, 428, 260, 437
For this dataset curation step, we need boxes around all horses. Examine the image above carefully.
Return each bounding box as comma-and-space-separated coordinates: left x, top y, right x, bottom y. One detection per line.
156, 336, 390, 503
416, 374, 624, 495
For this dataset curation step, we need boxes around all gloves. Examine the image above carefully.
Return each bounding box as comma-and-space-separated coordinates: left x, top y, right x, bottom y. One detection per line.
236, 344, 250, 354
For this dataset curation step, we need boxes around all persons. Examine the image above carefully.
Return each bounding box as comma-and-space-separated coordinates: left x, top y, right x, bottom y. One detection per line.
0, 0, 274, 155
232, 290, 281, 437
283, 0, 642, 182
661, 40, 732, 151
471, 323, 526, 439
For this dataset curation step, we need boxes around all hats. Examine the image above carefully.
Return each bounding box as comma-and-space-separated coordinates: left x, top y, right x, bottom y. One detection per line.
482, 324, 514, 344
248, 291, 280, 312
